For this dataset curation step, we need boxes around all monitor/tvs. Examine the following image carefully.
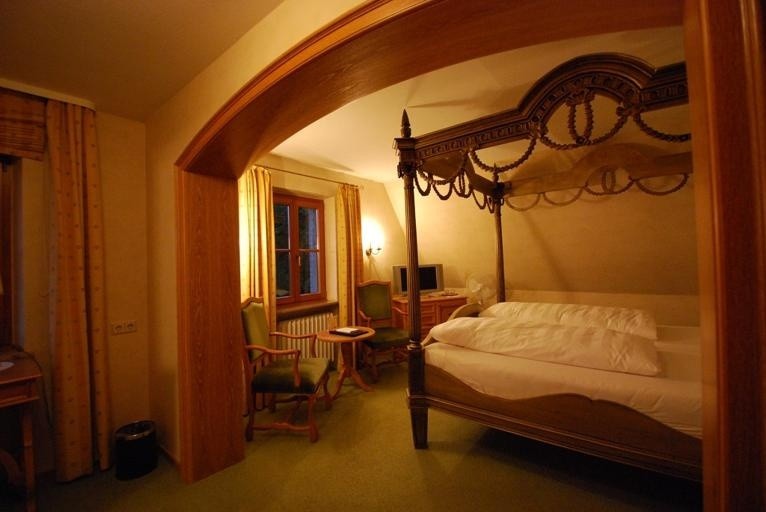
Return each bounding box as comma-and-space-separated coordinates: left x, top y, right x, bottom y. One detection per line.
392, 264, 444, 295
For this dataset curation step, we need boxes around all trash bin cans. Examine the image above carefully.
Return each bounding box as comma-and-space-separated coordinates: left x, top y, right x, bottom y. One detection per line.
113, 420, 159, 481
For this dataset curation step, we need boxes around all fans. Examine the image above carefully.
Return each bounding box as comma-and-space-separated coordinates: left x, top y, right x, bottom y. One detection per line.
465, 268, 497, 305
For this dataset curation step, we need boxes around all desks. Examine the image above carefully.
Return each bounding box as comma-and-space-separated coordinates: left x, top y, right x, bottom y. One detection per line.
0, 356, 43, 512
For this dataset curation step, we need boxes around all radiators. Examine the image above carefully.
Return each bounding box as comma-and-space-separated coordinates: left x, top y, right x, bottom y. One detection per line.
275, 312, 336, 364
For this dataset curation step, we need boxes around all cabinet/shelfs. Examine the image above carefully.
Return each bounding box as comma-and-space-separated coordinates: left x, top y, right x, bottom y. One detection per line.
392, 297, 467, 355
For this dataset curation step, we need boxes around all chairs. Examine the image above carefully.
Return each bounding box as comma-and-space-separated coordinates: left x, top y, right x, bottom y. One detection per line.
355, 280, 412, 384
239, 296, 333, 442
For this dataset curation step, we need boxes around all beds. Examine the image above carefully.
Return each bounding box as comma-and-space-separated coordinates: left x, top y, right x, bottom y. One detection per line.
391, 52, 702, 483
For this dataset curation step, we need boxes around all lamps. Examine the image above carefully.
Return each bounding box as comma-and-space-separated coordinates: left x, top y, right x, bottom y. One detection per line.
366, 239, 382, 256
